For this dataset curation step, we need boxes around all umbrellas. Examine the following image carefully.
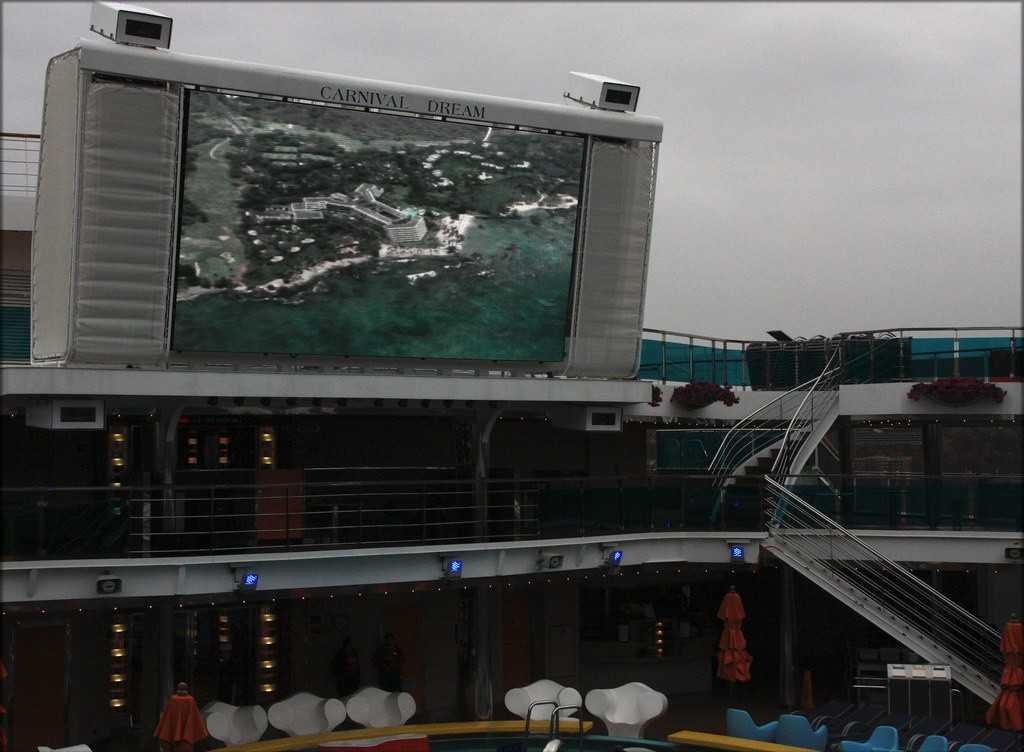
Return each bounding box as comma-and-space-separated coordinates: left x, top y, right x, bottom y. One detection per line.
987, 613, 1024, 733
715, 584, 753, 702
154, 682, 209, 752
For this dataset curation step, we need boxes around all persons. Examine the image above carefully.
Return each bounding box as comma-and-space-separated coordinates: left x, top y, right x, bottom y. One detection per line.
375, 632, 406, 693
336, 637, 360, 692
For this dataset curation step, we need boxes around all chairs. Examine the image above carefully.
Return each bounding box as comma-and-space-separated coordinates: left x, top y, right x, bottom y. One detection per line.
586, 681, 668, 738
341, 686, 416, 729
727, 707, 1022, 752
201, 701, 268, 747
505, 679, 583, 721
267, 692, 347, 737
38, 744, 92, 752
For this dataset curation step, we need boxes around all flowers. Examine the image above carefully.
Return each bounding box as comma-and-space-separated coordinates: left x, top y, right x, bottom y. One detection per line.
907, 376, 1007, 405
670, 380, 739, 407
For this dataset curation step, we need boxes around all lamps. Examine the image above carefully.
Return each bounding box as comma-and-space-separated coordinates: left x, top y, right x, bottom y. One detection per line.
286, 397, 298, 406
337, 398, 497, 411
233, 573, 260, 593
439, 559, 462, 580
261, 397, 271, 406
311, 397, 321, 406
731, 546, 746, 562
207, 396, 218, 406
600, 550, 623, 568
233, 397, 245, 406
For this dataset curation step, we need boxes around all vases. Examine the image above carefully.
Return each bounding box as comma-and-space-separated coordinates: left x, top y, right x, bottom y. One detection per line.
934, 397, 983, 407
681, 400, 712, 410
